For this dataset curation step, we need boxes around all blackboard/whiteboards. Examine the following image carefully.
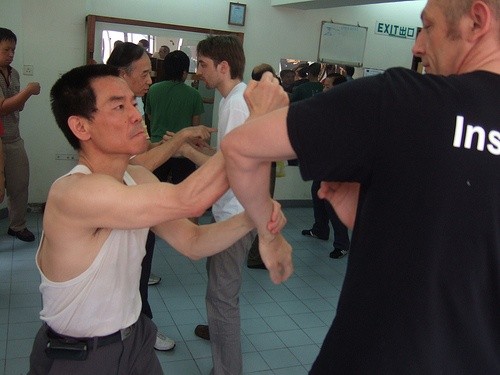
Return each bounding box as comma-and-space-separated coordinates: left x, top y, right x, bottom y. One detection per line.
318, 20, 368, 68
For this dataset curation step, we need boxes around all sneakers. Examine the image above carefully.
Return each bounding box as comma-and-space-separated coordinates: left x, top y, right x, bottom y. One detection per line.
154, 329, 176, 351
330, 248, 351, 259
302, 230, 323, 239
148, 274, 161, 285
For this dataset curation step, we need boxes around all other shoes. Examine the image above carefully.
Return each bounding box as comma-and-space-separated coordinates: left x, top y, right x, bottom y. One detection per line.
195, 325, 210, 340
247, 259, 266, 269
8, 227, 35, 242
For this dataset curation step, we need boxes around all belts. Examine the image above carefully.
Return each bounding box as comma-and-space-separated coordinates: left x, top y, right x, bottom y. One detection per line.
45, 324, 135, 349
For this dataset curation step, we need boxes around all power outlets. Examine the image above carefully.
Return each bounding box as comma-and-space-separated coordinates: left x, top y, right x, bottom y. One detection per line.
23, 65, 33, 75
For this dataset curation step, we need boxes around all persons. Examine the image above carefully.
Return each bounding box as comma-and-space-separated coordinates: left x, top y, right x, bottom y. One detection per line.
107, 40, 218, 352
164, 34, 258, 375
301, 73, 351, 258
221, 0, 500, 375
27, 65, 289, 375
0, 28, 42, 243
280, 64, 356, 101
